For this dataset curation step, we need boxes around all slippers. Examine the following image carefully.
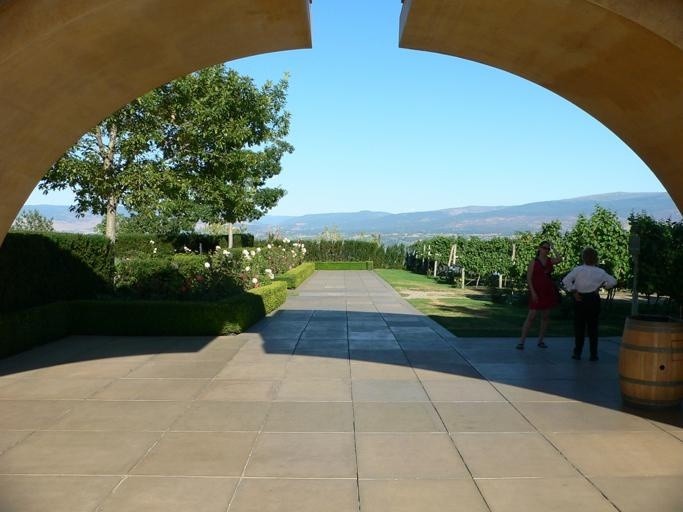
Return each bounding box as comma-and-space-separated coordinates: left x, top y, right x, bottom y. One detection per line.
517, 343, 523, 349
537, 342, 547, 348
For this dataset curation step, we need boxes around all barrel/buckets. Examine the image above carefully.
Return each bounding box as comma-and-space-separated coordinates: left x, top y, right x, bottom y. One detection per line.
618, 314, 683, 410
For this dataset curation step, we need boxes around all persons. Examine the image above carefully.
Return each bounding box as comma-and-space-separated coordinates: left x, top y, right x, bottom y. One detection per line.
561, 247, 618, 361
515, 240, 563, 350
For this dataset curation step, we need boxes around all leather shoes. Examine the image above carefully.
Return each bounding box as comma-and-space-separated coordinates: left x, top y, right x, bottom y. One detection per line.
590, 356, 598, 361
572, 355, 580, 360
